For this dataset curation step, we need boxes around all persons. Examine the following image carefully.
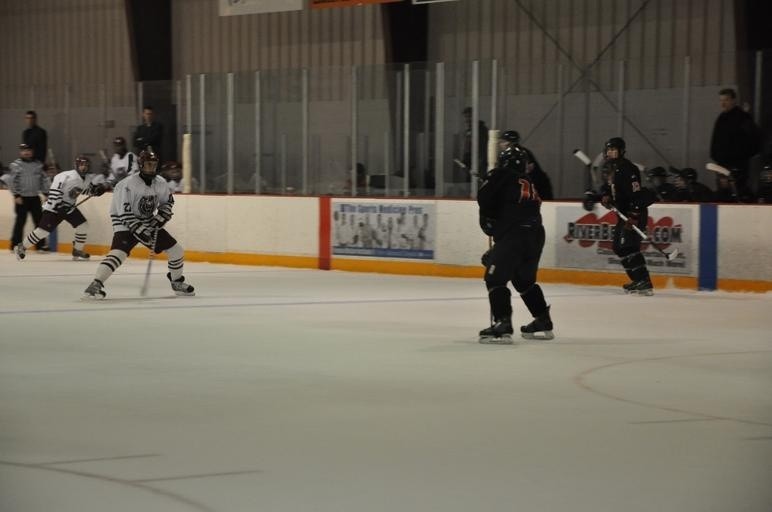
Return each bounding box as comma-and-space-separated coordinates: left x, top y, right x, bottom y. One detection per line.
80, 153, 195, 296
479, 157, 556, 339
343, 163, 368, 196
0, 139, 203, 259
495, 131, 553, 201
15, 112, 48, 176
131, 107, 162, 157
455, 109, 497, 194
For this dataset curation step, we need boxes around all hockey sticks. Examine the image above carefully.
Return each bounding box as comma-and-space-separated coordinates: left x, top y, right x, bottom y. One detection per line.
632, 161, 667, 202
609, 205, 678, 260
593, 150, 606, 194
574, 149, 592, 195
706, 163, 742, 203
99, 152, 118, 179
75, 154, 133, 209
139, 228, 157, 295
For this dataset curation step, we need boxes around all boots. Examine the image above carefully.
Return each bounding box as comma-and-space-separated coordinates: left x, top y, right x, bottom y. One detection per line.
478, 314, 513, 337
622, 270, 653, 291
520, 305, 554, 334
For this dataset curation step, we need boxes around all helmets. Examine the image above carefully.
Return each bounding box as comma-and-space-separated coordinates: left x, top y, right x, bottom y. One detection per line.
605, 137, 625, 151
73, 157, 91, 171
137, 149, 159, 168
500, 148, 529, 175
112, 136, 126, 148
499, 130, 520, 143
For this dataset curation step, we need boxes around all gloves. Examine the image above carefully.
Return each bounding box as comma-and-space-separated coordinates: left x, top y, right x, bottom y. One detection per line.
91, 181, 109, 197
128, 220, 155, 250
56, 200, 76, 216
583, 195, 594, 211
622, 210, 641, 234
150, 210, 173, 230
600, 194, 616, 210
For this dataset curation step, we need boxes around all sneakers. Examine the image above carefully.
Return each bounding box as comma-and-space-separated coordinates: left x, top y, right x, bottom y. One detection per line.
36, 245, 52, 251
85, 279, 107, 298
13, 243, 27, 259
71, 240, 90, 258
165, 272, 195, 292
